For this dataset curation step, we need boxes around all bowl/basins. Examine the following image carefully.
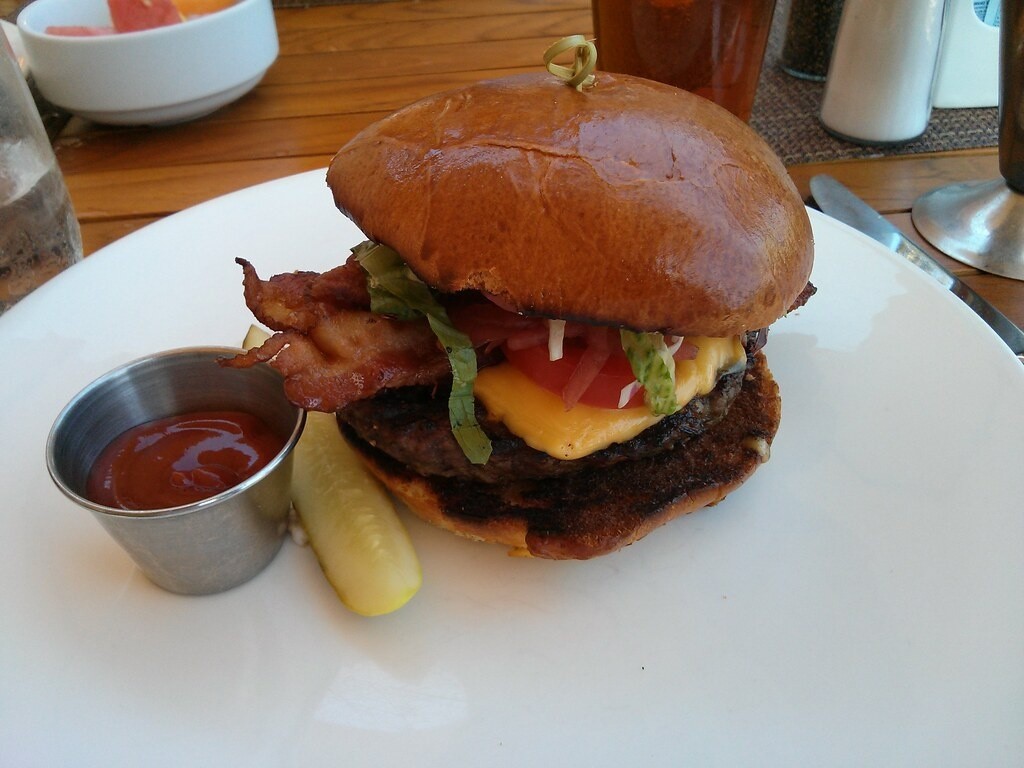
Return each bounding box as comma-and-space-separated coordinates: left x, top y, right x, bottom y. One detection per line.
44, 345, 310, 601
15, 0, 282, 127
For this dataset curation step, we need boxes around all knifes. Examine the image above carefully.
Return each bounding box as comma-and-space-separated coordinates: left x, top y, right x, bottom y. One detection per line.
802, 168, 1024, 355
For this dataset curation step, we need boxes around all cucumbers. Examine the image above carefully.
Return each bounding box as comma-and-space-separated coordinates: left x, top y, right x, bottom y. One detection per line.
240, 324, 421, 616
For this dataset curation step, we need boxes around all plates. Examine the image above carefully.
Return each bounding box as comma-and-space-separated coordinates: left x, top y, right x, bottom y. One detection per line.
0, 21, 29, 76
0, 167, 1024, 768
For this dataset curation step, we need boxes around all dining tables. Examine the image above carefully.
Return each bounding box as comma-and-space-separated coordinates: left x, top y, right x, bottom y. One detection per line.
0, 165, 1023, 768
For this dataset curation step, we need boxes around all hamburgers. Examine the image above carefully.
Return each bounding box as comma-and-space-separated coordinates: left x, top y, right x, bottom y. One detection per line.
236, 68, 817, 560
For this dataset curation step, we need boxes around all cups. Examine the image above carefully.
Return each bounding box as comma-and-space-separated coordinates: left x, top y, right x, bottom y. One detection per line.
912, 0, 1024, 285
0, 28, 84, 318
584, 0, 776, 126
780, 1, 847, 83
821, 0, 944, 143
